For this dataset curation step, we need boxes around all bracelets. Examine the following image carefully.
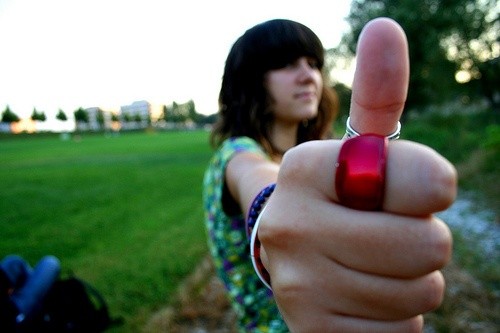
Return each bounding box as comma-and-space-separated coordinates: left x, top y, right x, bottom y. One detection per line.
246, 183, 279, 292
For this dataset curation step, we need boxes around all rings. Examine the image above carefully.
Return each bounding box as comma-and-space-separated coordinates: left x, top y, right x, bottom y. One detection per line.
336, 134, 390, 212
344, 116, 401, 141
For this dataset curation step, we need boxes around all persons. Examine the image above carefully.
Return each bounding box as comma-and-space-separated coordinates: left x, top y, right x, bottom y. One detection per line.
202, 16, 458, 333
